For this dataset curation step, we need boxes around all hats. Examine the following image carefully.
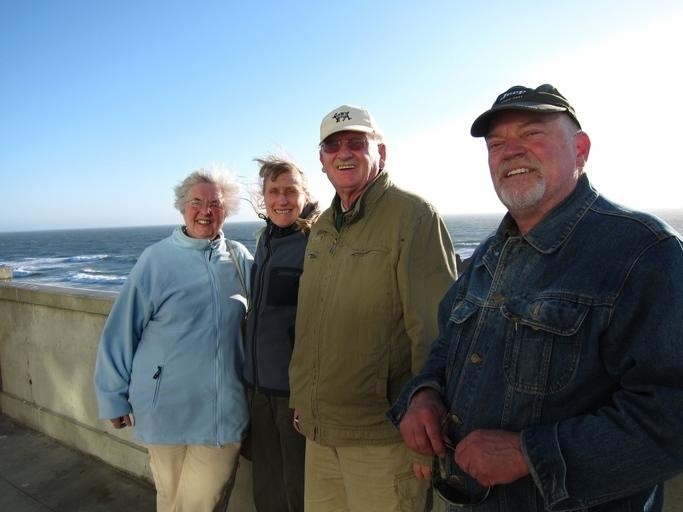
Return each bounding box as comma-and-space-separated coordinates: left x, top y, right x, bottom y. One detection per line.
470, 83, 583, 138
318, 103, 382, 147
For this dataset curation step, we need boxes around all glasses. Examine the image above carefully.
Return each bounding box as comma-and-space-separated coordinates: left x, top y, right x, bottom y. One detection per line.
190, 199, 223, 210
321, 135, 367, 153
429, 441, 492, 509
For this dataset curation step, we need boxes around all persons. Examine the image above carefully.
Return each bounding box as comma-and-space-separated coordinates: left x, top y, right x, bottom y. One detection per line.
287, 104, 460, 512
91, 167, 255, 510
239, 153, 323, 511
384, 82, 682, 512
